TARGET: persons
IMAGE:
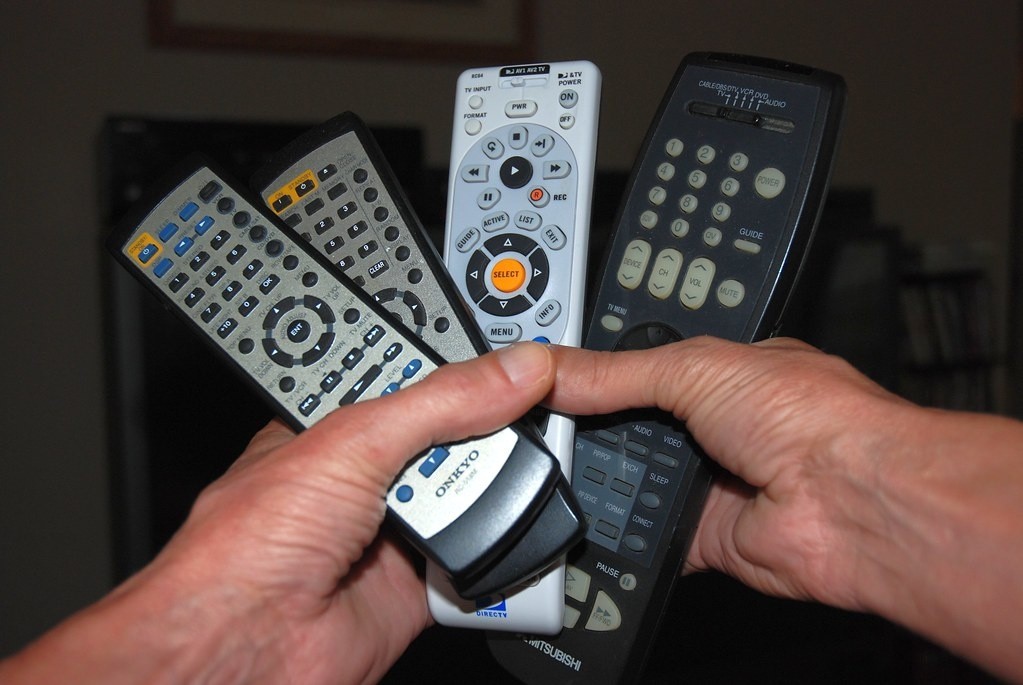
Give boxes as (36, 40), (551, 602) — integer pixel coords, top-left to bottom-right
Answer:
(0, 338), (1023, 685)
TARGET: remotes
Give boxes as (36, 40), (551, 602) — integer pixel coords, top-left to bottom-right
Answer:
(119, 50), (849, 685)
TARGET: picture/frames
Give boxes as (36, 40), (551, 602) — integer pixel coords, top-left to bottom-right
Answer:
(97, 116), (425, 584)
(146, 0), (541, 67)
